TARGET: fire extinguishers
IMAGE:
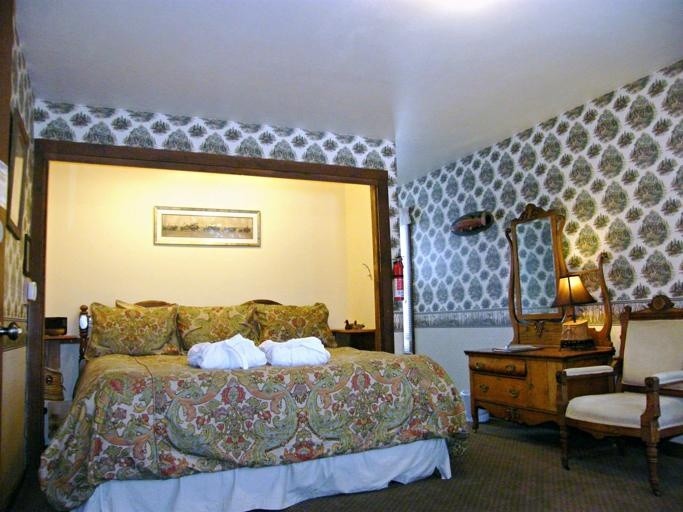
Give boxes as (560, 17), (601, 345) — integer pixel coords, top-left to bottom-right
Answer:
(392, 256), (404, 301)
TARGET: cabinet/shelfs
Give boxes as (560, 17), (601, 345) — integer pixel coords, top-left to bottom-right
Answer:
(463, 345), (615, 431)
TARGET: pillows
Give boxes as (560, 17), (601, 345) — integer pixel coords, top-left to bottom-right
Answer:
(113, 299), (177, 311)
(251, 300), (338, 347)
(82, 302), (181, 358)
(177, 299), (259, 351)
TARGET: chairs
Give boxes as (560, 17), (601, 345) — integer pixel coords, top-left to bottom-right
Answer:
(555, 293), (682, 496)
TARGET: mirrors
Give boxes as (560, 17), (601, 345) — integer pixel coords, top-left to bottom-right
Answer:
(510, 205), (563, 319)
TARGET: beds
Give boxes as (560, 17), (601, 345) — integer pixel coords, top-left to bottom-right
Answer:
(38, 299), (471, 510)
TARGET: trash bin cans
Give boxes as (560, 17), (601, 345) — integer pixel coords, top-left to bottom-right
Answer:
(461, 390), (490, 422)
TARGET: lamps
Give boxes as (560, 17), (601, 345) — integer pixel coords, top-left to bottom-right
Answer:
(550, 276), (597, 349)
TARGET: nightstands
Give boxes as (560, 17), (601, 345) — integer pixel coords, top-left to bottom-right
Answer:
(329, 327), (375, 350)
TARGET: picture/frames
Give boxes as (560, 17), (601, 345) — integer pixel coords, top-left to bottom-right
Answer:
(22, 234), (30, 276)
(5, 109), (30, 239)
(153, 205), (261, 247)
